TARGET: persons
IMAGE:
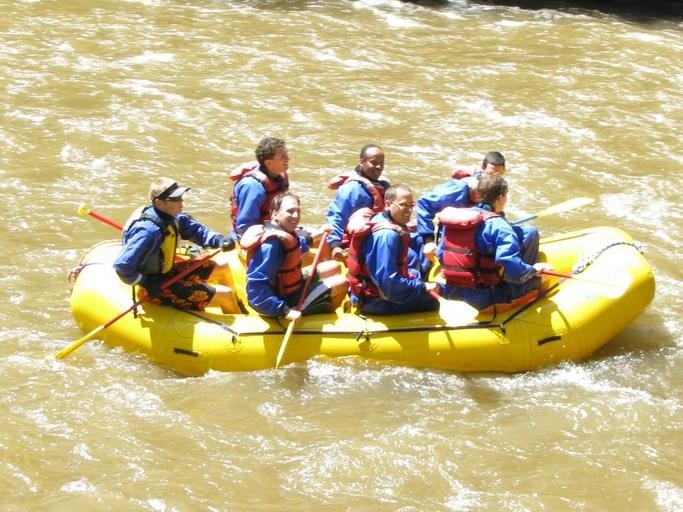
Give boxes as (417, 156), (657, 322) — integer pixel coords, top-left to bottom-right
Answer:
(346, 185), (439, 316)
(325, 145), (393, 261)
(247, 191), (336, 323)
(114, 177), (235, 310)
(230, 138), (290, 247)
(432, 175), (543, 310)
(417, 151), (540, 265)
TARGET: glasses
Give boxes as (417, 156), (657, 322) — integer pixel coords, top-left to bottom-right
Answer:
(392, 200), (416, 210)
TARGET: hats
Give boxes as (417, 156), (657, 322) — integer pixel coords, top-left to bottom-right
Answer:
(150, 177), (190, 201)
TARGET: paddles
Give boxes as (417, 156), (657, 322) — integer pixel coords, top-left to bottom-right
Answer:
(276, 225), (330, 370)
(427, 288), (479, 328)
(512, 197), (597, 225)
(539, 266), (635, 300)
(55, 234), (242, 360)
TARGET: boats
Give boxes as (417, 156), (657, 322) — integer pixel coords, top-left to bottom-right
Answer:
(66, 223), (657, 375)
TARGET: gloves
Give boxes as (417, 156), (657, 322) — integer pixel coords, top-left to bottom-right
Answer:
(140, 273), (165, 298)
(216, 237), (235, 252)
